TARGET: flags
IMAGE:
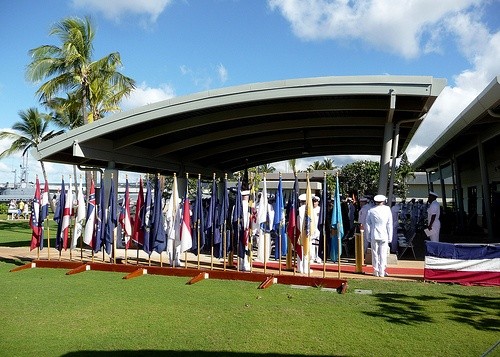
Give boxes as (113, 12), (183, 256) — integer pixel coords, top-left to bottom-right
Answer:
(53, 178), (74, 252)
(192, 178), (244, 259)
(248, 176), (344, 264)
(118, 177), (192, 266)
(29, 179), (49, 252)
(70, 178), (118, 254)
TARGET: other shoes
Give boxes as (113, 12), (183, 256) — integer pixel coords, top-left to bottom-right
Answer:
(240, 264), (250, 271)
(379, 273), (388, 277)
(375, 272), (379, 276)
(300, 269), (313, 273)
(171, 262), (183, 266)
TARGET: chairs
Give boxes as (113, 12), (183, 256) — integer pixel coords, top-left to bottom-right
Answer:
(396, 233), (417, 259)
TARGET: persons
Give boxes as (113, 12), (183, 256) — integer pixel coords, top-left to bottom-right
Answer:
(310, 196), (322, 264)
(252, 191), (275, 263)
(390, 199), (426, 253)
(51, 195), (57, 201)
(296, 193), (317, 273)
(7, 198), (28, 220)
(359, 194), (393, 277)
(425, 192), (440, 242)
(231, 190), (257, 272)
(341, 196), (355, 237)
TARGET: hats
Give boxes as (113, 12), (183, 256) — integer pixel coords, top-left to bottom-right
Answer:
(240, 190), (250, 196)
(298, 193), (307, 202)
(179, 198), (182, 204)
(314, 196), (320, 201)
(258, 192), (262, 196)
(374, 194), (386, 203)
(428, 191), (438, 197)
(360, 198), (367, 202)
